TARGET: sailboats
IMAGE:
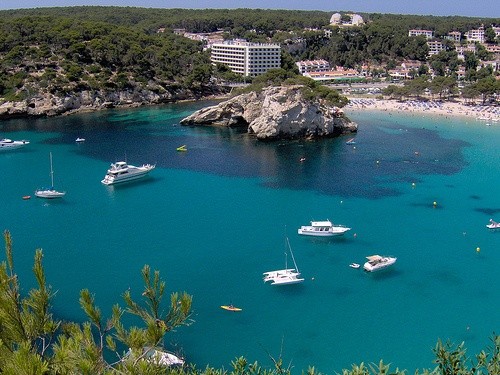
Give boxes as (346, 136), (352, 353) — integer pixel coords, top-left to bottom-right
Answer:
(260, 225), (305, 285)
(35, 152), (66, 198)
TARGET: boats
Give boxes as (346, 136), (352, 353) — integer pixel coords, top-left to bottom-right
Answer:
(299, 157), (306, 162)
(346, 137), (356, 145)
(101, 160), (156, 185)
(363, 254), (398, 272)
(22, 196), (31, 200)
(485, 224), (500, 229)
(120, 346), (188, 369)
(76, 137), (85, 141)
(221, 305), (243, 312)
(348, 262), (361, 269)
(296, 220), (351, 237)
(176, 145), (188, 151)
(0, 138), (30, 149)
